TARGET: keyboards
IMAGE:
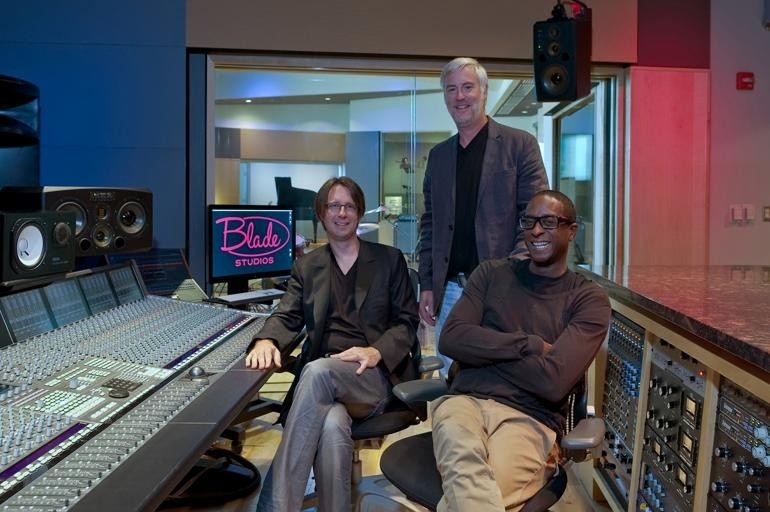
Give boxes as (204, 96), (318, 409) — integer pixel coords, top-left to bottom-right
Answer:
(216, 287), (286, 302)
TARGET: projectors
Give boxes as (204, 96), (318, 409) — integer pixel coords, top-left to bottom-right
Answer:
(532, 6), (592, 103)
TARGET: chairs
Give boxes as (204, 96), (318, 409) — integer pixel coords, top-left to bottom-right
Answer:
(274, 268), (443, 512)
(380, 374), (606, 512)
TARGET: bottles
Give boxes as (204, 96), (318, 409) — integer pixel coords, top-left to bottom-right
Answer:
(296, 233), (304, 261)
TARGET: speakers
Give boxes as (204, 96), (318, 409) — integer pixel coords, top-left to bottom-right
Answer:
(0, 211), (77, 282)
(41, 183), (155, 254)
(215, 126), (241, 158)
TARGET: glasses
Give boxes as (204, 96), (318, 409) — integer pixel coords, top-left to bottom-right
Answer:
(326, 203), (357, 212)
(518, 215), (572, 230)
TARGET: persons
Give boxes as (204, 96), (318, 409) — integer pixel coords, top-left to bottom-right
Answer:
(429, 190), (612, 512)
(418, 56), (551, 385)
(243, 175), (423, 511)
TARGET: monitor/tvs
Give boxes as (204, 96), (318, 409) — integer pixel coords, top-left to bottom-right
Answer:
(207, 204), (298, 295)
